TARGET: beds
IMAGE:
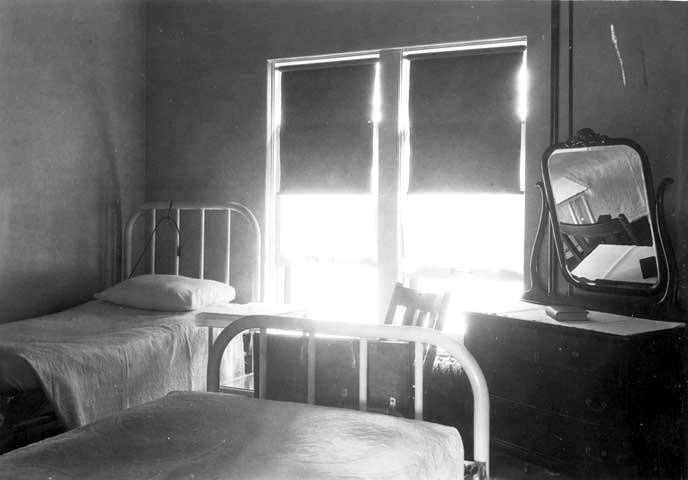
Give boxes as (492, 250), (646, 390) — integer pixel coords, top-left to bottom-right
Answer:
(0, 202), (260, 453)
(0, 314), (490, 480)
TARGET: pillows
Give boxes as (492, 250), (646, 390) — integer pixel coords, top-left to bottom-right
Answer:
(94, 273), (235, 312)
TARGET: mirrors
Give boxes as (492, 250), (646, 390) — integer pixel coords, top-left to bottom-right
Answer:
(519, 129), (674, 318)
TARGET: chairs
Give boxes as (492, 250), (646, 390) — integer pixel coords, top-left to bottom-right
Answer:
(378, 283), (445, 400)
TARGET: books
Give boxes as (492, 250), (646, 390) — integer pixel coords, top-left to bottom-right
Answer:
(640, 256), (658, 279)
(546, 305), (588, 321)
